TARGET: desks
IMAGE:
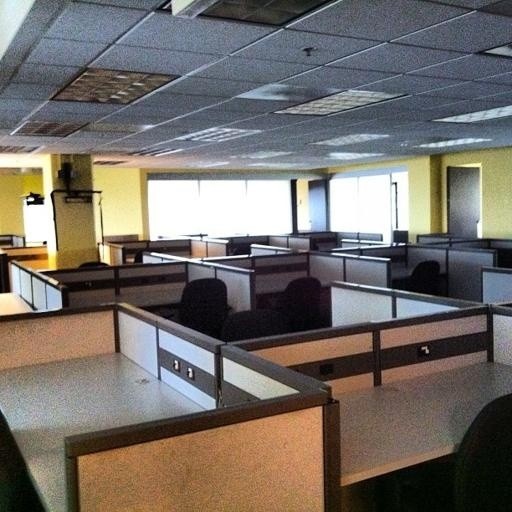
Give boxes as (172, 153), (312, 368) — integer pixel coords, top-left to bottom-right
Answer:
(0, 231), (512, 512)
